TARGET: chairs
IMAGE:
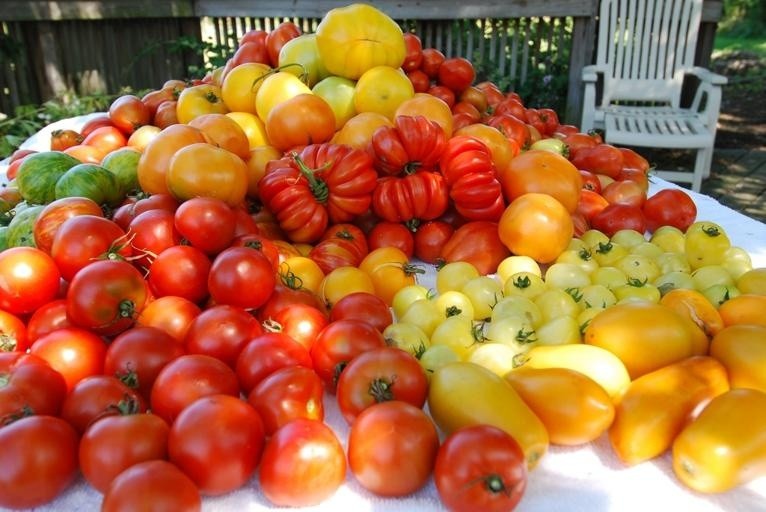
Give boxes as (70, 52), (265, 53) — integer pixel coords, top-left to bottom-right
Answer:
(581, 1), (729, 194)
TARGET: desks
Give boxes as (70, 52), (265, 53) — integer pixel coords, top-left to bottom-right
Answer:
(0, 110), (766, 511)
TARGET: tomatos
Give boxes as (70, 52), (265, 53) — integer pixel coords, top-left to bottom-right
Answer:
(0, 4), (766, 512)
